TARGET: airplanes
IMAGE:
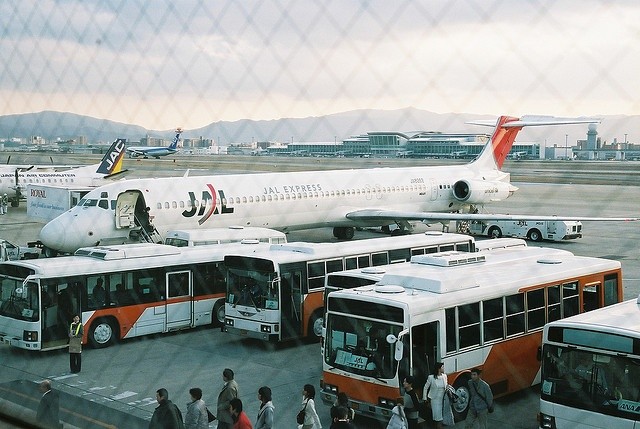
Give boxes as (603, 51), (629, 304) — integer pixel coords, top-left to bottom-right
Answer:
(40, 116), (640, 253)
(0, 139), (126, 207)
(125, 133), (180, 159)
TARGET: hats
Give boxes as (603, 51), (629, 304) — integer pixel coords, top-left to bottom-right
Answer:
(259, 385), (272, 400)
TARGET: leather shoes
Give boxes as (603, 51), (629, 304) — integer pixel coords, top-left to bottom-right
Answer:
(70, 369), (80, 373)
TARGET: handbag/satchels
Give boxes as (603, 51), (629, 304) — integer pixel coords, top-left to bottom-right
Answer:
(472, 382), (494, 413)
(205, 407), (217, 423)
(296, 396), (314, 424)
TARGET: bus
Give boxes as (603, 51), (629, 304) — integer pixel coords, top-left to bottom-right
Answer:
(539, 295), (640, 429)
(321, 246), (574, 357)
(0, 240), (278, 352)
(319, 255), (623, 427)
(163, 226), (287, 246)
(467, 215), (583, 243)
(224, 232), (476, 344)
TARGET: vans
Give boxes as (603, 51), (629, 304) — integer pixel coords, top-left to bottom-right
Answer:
(475, 238), (526, 252)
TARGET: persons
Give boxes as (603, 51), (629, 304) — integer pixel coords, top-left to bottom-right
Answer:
(216, 368), (238, 429)
(93, 277), (105, 302)
(295, 385), (323, 429)
(36, 382), (58, 427)
(115, 283), (123, 301)
(228, 398), (252, 428)
(3, 193), (8, 214)
(394, 375), (423, 429)
(330, 406), (354, 429)
(255, 387), (275, 429)
(148, 387), (183, 428)
(67, 316), (84, 373)
(183, 388), (209, 429)
(423, 362), (449, 429)
(0, 195), (3, 215)
(330, 392), (355, 429)
(139, 207), (151, 231)
(464, 369), (495, 428)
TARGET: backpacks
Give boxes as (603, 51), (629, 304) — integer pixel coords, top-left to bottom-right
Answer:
(348, 404), (355, 422)
